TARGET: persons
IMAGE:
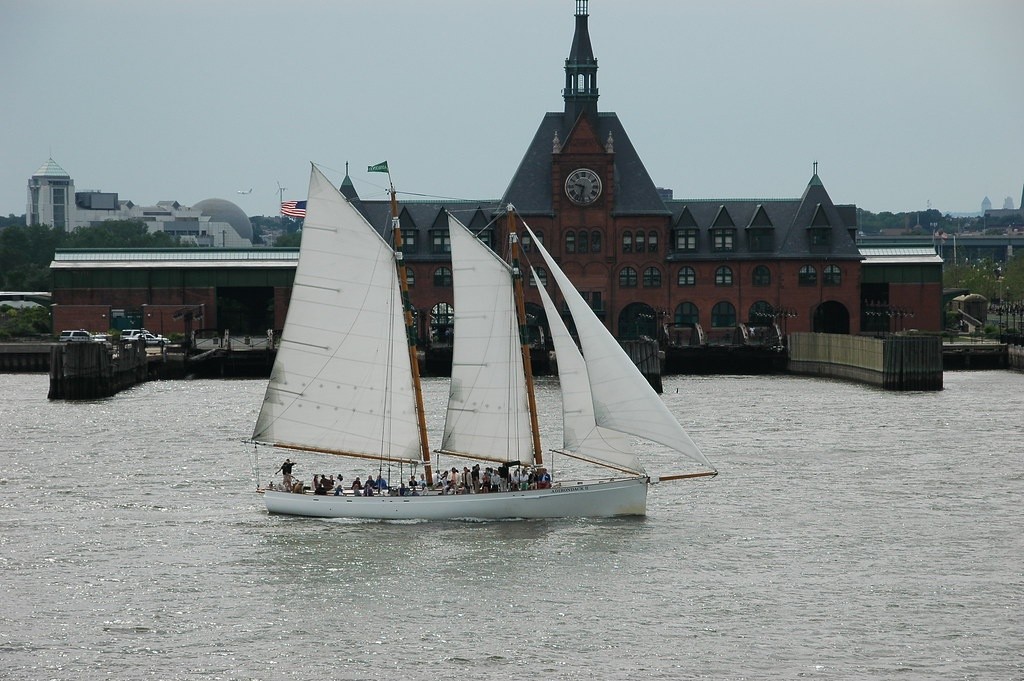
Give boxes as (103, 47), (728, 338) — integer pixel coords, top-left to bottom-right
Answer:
(429, 325), (454, 347)
(989, 302), (1024, 316)
(275, 459), (297, 493)
(311, 463), (552, 497)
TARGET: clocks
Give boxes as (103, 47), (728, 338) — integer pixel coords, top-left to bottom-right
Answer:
(565, 169), (602, 205)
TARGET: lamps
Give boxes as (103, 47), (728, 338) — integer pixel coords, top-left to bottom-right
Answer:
(147, 313), (152, 317)
(102, 314), (106, 317)
(49, 312), (54, 316)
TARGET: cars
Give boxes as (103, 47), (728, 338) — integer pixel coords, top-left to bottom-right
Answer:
(127, 333), (172, 347)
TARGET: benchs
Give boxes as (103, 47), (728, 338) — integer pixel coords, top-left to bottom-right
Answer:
(305, 487), (468, 496)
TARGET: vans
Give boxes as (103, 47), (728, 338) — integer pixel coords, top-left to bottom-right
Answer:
(119, 327), (151, 343)
(59, 329), (94, 344)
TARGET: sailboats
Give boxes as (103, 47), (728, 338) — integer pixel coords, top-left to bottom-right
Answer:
(250, 161), (719, 519)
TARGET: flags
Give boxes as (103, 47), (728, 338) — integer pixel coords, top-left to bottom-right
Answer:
(368, 162), (388, 174)
(280, 200), (308, 219)
